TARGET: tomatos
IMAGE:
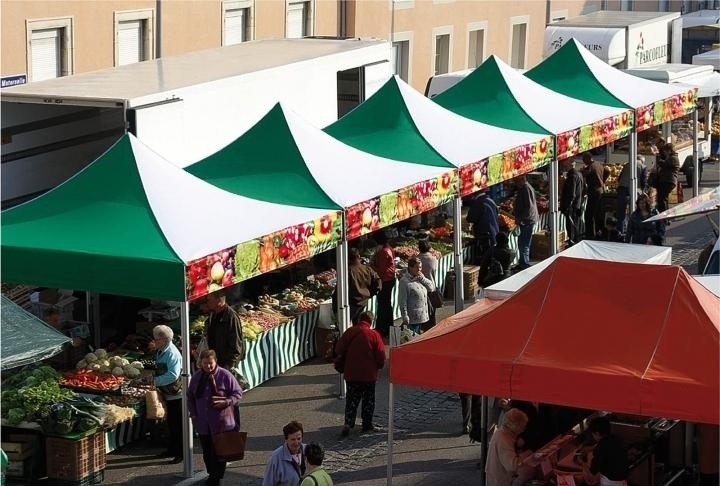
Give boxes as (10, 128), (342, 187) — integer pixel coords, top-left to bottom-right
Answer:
(279, 245), (288, 258)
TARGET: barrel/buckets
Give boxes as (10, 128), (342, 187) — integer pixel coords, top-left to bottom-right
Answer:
(313, 323), (339, 359)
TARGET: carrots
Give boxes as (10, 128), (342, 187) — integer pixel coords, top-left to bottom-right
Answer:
(62, 369), (127, 391)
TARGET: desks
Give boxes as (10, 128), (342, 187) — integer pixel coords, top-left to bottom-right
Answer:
(188, 286), (321, 390)
(285, 180), (587, 334)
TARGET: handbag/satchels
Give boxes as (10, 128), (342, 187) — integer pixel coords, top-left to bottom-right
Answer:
(211, 432), (248, 463)
(482, 247), (504, 284)
(427, 287), (444, 308)
(144, 376), (168, 420)
(334, 352), (346, 374)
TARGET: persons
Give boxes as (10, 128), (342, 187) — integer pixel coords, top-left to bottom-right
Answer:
(206, 291), (245, 432)
(399, 256), (435, 341)
(332, 248), (382, 325)
(698, 239), (715, 273)
(186, 349), (242, 485)
(418, 241), (437, 331)
(66, 335), (86, 369)
(334, 310), (386, 435)
(298, 442), (333, 486)
(697, 98), (707, 123)
(466, 138), (679, 287)
(458, 394), (627, 486)
(372, 229), (397, 343)
(714, 96), (720, 113)
(153, 325), (184, 464)
(263, 421), (307, 485)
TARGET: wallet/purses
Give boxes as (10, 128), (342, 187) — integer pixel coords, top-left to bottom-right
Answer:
(209, 395), (226, 407)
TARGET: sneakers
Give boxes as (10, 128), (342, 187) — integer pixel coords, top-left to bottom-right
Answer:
(160, 450), (183, 464)
(342, 425), (380, 438)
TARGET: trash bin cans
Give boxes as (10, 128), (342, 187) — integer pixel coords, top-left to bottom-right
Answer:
(683, 156), (704, 187)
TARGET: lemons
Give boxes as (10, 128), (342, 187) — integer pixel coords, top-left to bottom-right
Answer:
(644, 111), (650, 123)
(362, 208), (372, 228)
(567, 137), (574, 151)
(474, 170), (481, 186)
(211, 262), (224, 281)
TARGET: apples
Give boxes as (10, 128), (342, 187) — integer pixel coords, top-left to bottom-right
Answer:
(351, 222), (361, 231)
(193, 279), (207, 296)
(222, 269), (233, 287)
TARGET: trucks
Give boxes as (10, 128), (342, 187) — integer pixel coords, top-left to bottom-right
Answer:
(541, 7), (685, 67)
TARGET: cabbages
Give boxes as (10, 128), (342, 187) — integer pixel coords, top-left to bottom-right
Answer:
(76, 348), (144, 378)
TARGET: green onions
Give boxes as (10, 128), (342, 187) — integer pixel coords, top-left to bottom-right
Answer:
(63, 396), (136, 427)
